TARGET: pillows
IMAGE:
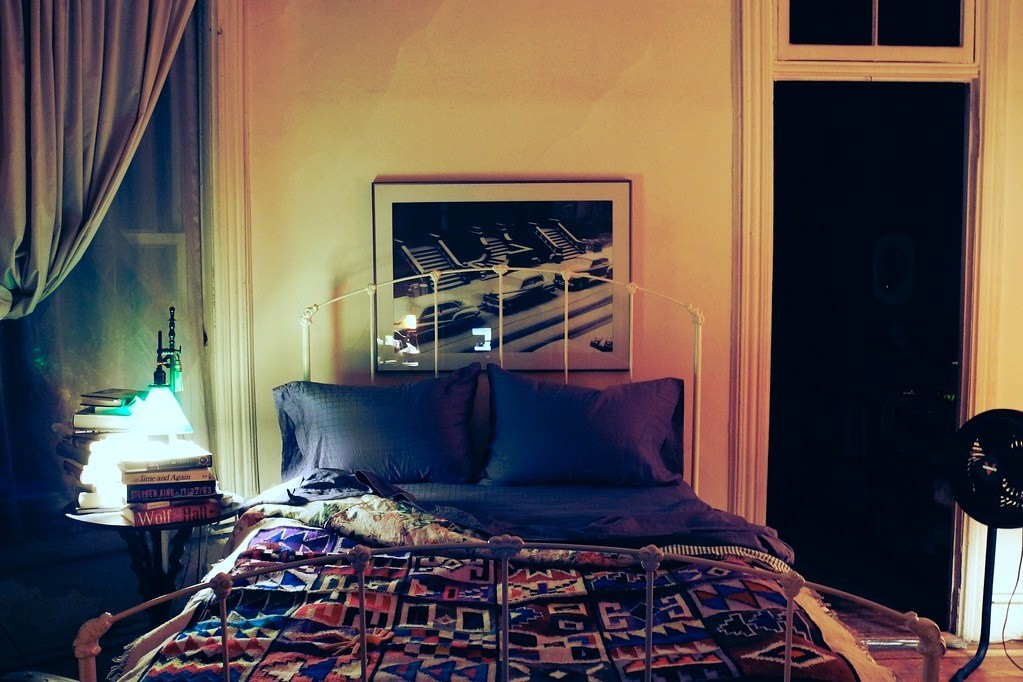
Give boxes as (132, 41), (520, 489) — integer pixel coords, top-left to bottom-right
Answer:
(480, 364), (684, 488)
(273, 362), (481, 484)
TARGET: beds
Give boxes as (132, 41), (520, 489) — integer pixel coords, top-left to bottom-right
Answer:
(72, 263), (947, 682)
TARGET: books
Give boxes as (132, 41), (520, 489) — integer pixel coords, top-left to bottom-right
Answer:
(56, 387), (240, 527)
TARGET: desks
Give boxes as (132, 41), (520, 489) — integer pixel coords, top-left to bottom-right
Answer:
(66, 491), (243, 631)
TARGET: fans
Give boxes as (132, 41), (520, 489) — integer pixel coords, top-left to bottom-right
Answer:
(948, 409), (1023, 682)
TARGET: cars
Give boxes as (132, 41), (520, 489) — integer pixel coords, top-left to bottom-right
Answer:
(395, 300), (481, 346)
(553, 256), (613, 292)
(482, 270), (554, 314)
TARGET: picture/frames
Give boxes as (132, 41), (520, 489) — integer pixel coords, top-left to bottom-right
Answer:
(372, 180), (633, 374)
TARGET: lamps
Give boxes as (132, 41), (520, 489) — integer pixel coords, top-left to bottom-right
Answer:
(130, 307), (194, 436)
